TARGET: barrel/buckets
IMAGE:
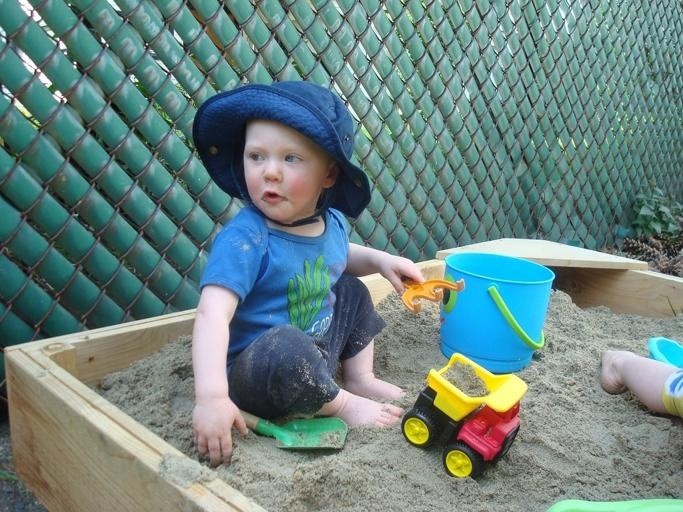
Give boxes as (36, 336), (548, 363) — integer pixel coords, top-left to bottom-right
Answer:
(439, 252), (558, 372)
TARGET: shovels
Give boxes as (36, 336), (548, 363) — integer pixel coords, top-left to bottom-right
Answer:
(230, 405), (348, 452)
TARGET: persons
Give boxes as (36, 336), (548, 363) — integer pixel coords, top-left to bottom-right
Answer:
(189, 83), (425, 466)
(597, 347), (682, 416)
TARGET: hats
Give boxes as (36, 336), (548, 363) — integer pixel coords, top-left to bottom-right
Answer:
(192, 80), (371, 218)
